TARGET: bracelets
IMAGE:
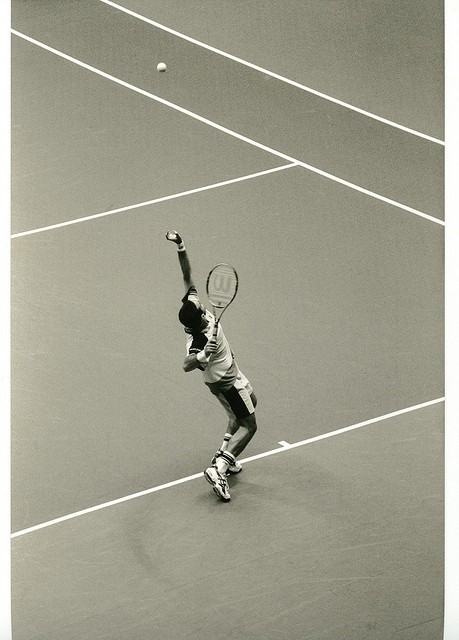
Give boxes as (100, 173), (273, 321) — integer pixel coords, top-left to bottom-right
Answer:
(177, 241), (184, 248)
(197, 350), (207, 363)
(176, 247), (185, 252)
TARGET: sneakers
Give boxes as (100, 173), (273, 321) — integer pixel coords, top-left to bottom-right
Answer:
(205, 466), (231, 502)
(211, 449), (242, 472)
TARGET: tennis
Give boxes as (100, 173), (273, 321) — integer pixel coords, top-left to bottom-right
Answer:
(157, 63), (166, 72)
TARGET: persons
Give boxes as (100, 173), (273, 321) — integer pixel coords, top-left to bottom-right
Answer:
(164, 230), (257, 501)
